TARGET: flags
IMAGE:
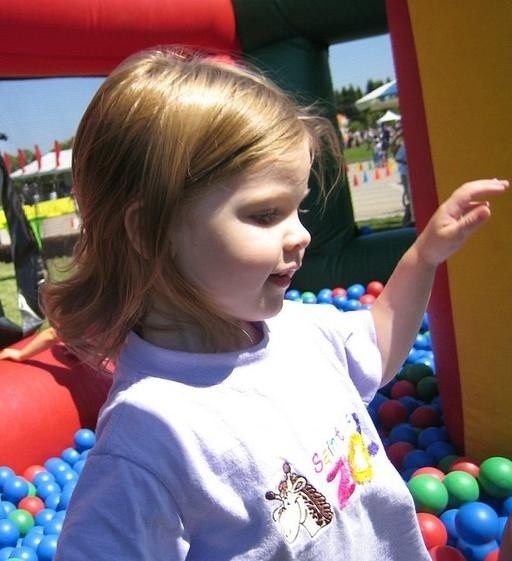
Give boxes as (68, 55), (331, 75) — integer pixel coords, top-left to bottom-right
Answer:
(18, 147), (26, 173)
(55, 139), (61, 167)
(34, 144), (42, 170)
(4, 151), (12, 176)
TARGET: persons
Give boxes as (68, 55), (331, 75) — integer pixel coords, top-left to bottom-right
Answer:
(343, 125), (411, 227)
(36, 42), (512, 561)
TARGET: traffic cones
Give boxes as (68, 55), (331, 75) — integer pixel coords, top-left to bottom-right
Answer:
(345, 159), (397, 187)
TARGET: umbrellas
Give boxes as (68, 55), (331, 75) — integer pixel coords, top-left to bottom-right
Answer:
(355, 79), (400, 112)
(375, 109), (401, 123)
